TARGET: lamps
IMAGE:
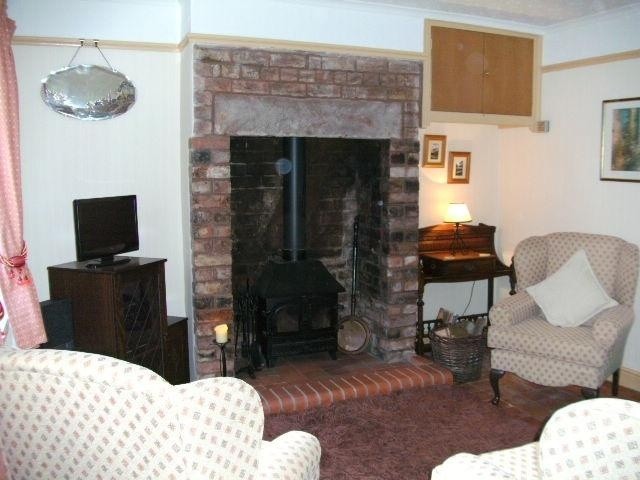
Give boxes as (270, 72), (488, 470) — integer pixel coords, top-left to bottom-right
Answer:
(443, 202), (473, 256)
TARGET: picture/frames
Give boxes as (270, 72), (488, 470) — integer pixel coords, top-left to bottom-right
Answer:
(448, 151), (471, 184)
(600, 97), (640, 183)
(422, 134), (447, 168)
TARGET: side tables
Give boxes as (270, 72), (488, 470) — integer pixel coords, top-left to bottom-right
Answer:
(416, 223), (517, 357)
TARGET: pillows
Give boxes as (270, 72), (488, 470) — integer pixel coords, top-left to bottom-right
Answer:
(525, 248), (620, 329)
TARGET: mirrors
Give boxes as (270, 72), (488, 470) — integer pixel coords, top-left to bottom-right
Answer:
(41, 63), (136, 120)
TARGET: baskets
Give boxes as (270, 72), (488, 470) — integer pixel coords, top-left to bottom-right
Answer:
(428, 324), (487, 384)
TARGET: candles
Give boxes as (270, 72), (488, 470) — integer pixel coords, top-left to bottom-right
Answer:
(213, 324), (229, 344)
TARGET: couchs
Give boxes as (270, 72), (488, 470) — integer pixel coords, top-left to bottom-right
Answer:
(487, 232), (639, 405)
(431, 398), (640, 480)
(0, 349), (323, 480)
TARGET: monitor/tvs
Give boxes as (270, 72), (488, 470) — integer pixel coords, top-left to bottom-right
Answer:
(73, 195), (140, 269)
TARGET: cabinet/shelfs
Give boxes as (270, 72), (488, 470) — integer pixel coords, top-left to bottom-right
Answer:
(47, 257), (190, 385)
(422, 18), (543, 128)
